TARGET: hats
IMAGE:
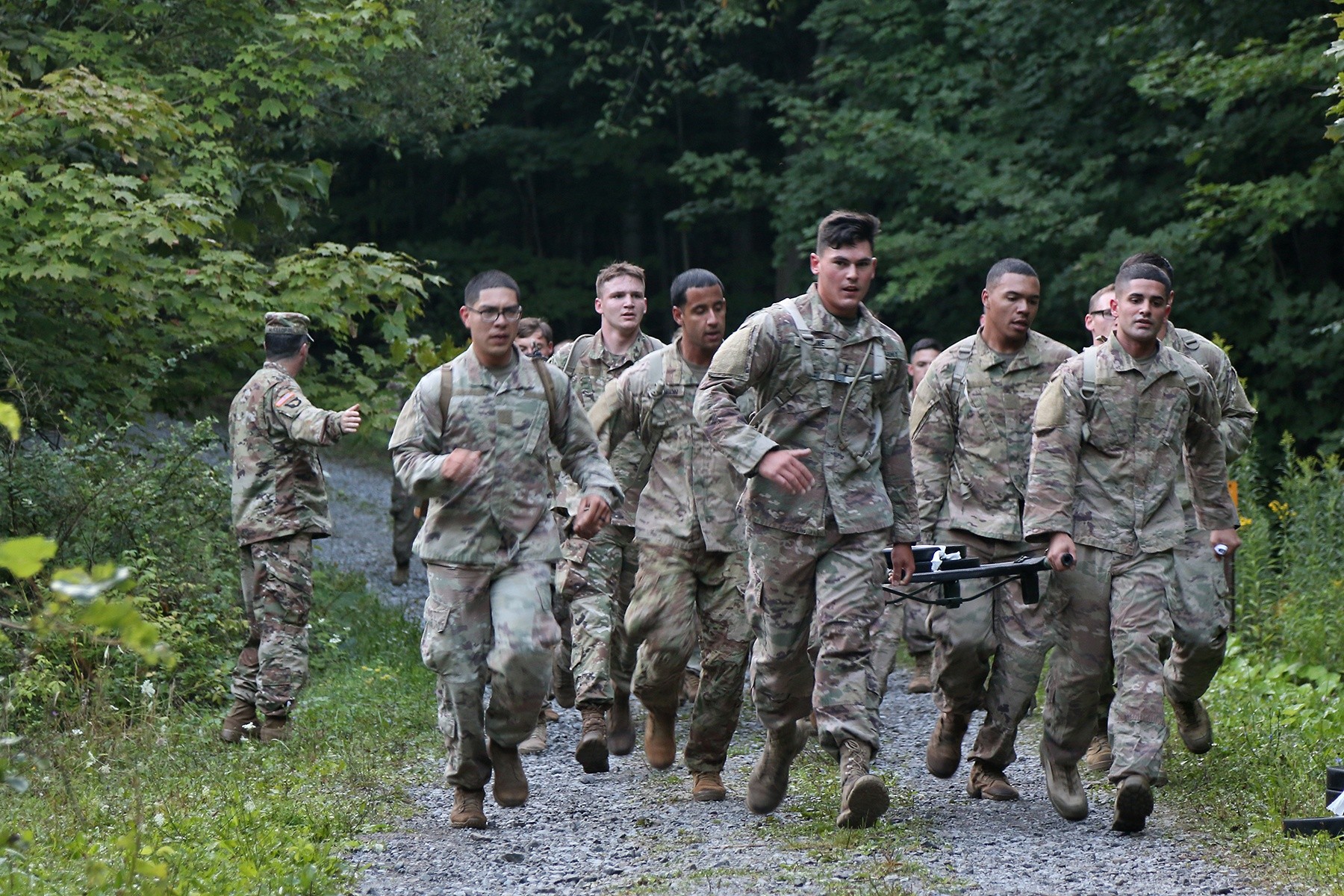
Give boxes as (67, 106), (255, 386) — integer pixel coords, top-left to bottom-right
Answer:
(265, 311), (314, 342)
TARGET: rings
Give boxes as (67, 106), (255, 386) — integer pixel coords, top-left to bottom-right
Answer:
(600, 517), (605, 522)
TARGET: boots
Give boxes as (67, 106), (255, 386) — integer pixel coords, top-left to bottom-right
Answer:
(607, 701), (636, 756)
(966, 762), (1020, 800)
(488, 738), (528, 807)
(261, 716), (322, 743)
(1164, 681), (1213, 754)
(927, 712), (971, 778)
(1040, 750), (1088, 823)
(836, 739), (889, 829)
(222, 700), (264, 743)
(1087, 722), (1114, 772)
(518, 711), (547, 753)
(574, 706), (610, 773)
(907, 650), (934, 693)
(451, 786), (487, 829)
(1111, 773), (1154, 833)
(551, 645), (576, 708)
(747, 720), (808, 814)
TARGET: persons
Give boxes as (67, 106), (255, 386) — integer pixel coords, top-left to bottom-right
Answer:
(386, 210), (1259, 833)
(220, 311), (362, 744)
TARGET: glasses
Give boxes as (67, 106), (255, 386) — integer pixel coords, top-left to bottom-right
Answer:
(466, 306), (522, 322)
(1090, 309), (1115, 320)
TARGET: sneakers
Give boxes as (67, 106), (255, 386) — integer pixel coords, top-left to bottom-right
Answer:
(685, 672), (699, 703)
(692, 772), (726, 801)
(645, 710), (676, 769)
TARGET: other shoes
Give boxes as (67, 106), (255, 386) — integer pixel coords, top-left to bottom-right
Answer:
(392, 565), (410, 584)
(804, 714), (817, 736)
